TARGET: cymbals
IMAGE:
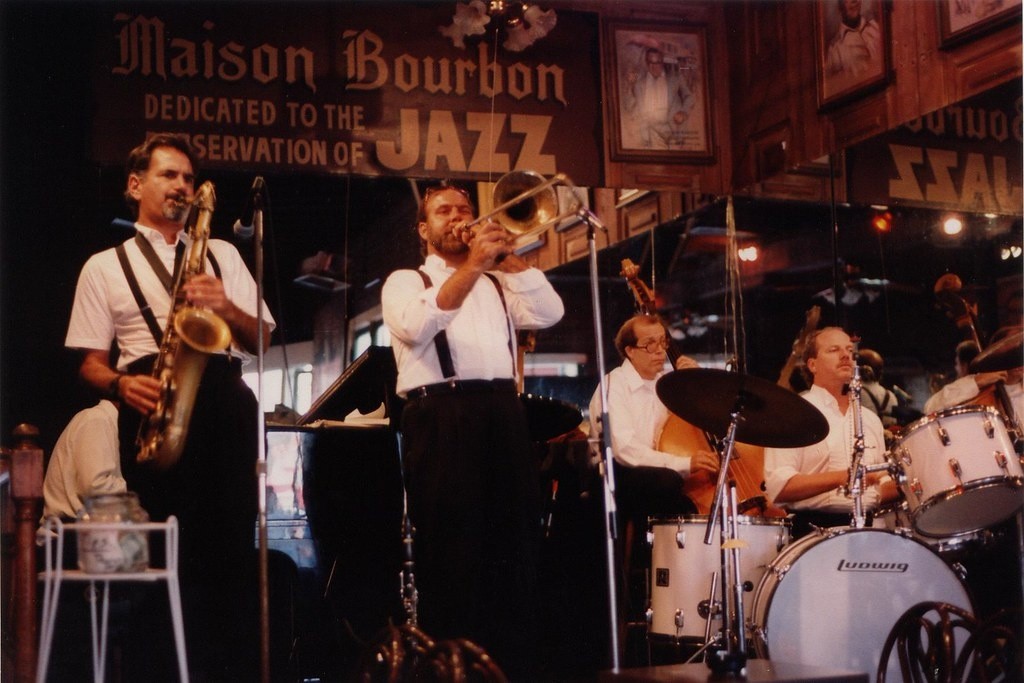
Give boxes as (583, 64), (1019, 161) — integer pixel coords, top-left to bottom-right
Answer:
(655, 367), (830, 449)
(971, 330), (1024, 364)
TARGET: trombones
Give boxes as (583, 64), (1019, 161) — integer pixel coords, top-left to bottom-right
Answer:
(459, 169), (583, 253)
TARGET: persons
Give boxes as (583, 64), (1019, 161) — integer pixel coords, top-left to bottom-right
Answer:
(382, 180), (566, 683)
(763, 326), (900, 541)
(63, 131), (276, 683)
(588, 316), (720, 611)
(855, 348), (899, 417)
(922, 325), (1024, 451)
(954, 340), (979, 377)
(36, 390), (154, 683)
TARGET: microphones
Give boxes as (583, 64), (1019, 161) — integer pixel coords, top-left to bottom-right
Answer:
(233, 176), (263, 239)
(569, 200), (607, 234)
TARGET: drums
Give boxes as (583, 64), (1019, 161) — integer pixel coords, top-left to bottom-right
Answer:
(750, 524), (981, 683)
(885, 400), (1024, 543)
(646, 514), (791, 642)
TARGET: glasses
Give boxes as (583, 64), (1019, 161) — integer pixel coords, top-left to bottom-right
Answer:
(424, 186), (471, 207)
(633, 338), (670, 354)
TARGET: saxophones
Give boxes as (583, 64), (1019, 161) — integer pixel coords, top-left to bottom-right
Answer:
(133, 181), (231, 464)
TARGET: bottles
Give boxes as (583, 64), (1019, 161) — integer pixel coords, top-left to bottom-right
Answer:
(75, 493), (150, 575)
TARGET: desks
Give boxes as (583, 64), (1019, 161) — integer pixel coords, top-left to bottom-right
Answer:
(34, 516), (192, 683)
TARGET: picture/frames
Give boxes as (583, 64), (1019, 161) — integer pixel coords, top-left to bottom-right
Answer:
(810, 0), (893, 114)
(613, 188), (651, 209)
(934, 0), (1023, 50)
(512, 232), (546, 255)
(601, 15), (718, 168)
(551, 185), (593, 232)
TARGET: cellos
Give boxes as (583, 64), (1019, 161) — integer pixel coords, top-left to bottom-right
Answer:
(621, 257), (787, 518)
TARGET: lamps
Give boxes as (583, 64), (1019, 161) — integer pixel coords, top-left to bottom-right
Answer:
(453, 0), (556, 52)
